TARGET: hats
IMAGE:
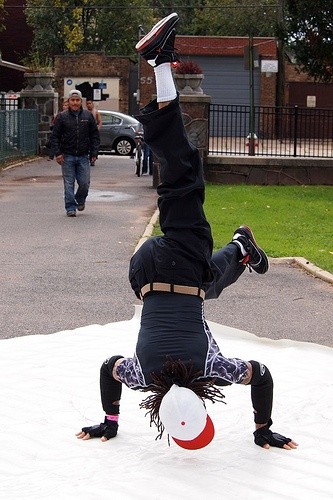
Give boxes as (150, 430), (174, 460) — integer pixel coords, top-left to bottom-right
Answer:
(158, 384), (215, 451)
(67, 89), (83, 101)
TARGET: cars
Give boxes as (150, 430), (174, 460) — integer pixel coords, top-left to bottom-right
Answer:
(96, 110), (144, 156)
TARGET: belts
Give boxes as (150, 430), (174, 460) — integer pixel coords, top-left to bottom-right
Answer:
(138, 283), (206, 301)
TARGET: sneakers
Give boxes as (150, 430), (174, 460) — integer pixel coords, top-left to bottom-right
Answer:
(135, 12), (182, 68)
(230, 225), (270, 275)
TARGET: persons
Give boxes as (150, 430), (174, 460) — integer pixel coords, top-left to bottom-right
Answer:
(75, 12), (297, 450)
(85, 98), (101, 167)
(137, 136), (154, 177)
(50, 100), (68, 128)
(52, 90), (100, 217)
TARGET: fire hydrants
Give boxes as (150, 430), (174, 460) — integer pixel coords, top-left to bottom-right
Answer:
(246, 133), (258, 156)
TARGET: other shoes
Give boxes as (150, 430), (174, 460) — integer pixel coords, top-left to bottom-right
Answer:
(48, 158), (52, 161)
(77, 202), (85, 211)
(90, 161), (96, 166)
(66, 210), (77, 217)
